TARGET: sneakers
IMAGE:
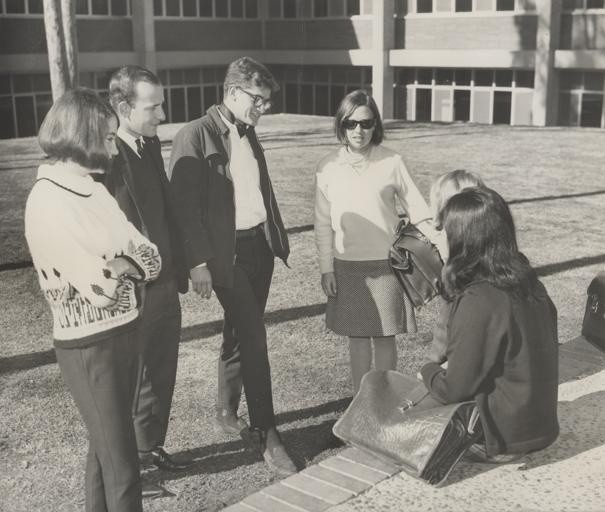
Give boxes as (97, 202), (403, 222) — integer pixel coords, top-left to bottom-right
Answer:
(249, 426), (297, 476)
(213, 415), (251, 437)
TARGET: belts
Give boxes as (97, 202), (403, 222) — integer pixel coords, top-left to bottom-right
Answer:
(235, 225), (265, 240)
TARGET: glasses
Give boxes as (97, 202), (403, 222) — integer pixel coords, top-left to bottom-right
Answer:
(344, 118), (377, 130)
(236, 86), (273, 109)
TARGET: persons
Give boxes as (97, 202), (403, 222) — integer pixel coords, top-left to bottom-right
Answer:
(414, 185), (562, 464)
(414, 168), (482, 382)
(24, 86), (164, 512)
(166, 55), (299, 477)
(89, 64), (191, 483)
(312, 89), (434, 399)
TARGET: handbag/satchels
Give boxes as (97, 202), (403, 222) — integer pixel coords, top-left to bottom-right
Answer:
(389, 220), (445, 311)
(333, 366), (485, 489)
(583, 270), (604, 352)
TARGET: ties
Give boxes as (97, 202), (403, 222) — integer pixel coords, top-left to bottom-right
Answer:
(135, 139), (144, 157)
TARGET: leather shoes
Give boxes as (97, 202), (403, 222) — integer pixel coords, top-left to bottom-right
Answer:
(140, 481), (165, 500)
(137, 448), (195, 471)
